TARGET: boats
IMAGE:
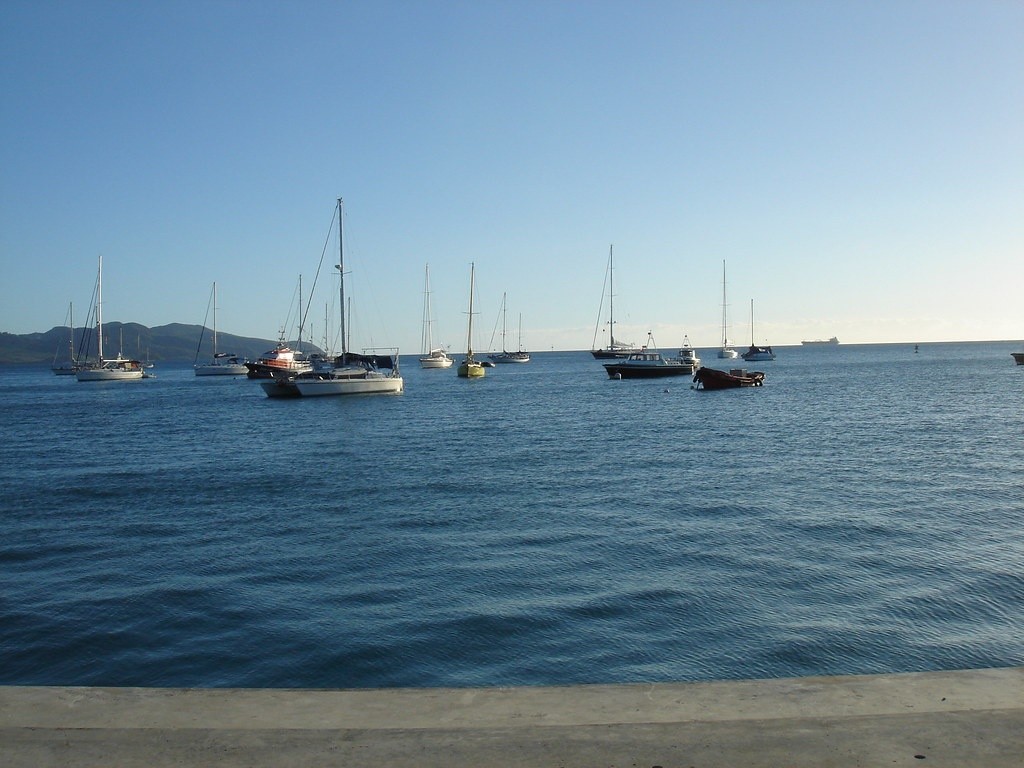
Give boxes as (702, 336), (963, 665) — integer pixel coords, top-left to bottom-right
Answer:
(693, 366), (765, 390)
(481, 361), (496, 367)
(674, 336), (701, 369)
(602, 329), (694, 380)
(1010, 352), (1024, 365)
(801, 337), (839, 345)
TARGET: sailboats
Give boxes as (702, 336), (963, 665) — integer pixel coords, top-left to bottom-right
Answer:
(51, 301), (80, 376)
(191, 281), (248, 376)
(418, 262), (453, 369)
(244, 274), (334, 379)
(259, 198), (404, 396)
(588, 243), (646, 359)
(457, 261), (485, 379)
(740, 299), (778, 361)
(75, 254), (146, 381)
(718, 259), (738, 359)
(486, 292), (530, 364)
(115, 327), (155, 369)
